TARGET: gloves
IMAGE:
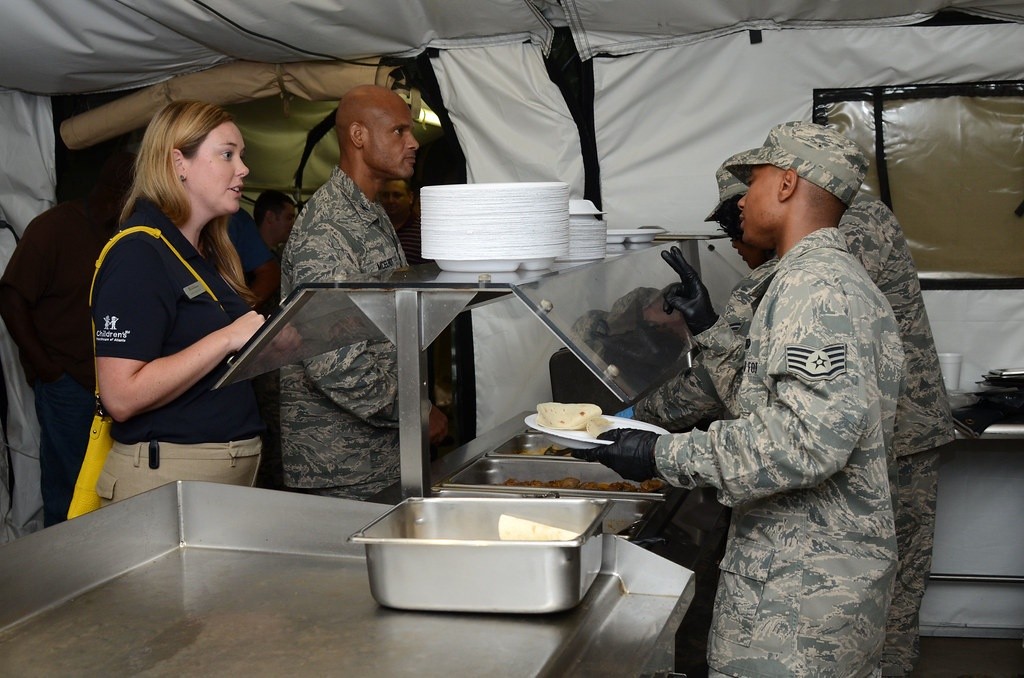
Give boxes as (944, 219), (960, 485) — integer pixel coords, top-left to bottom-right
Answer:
(660, 245), (720, 337)
(571, 428), (664, 482)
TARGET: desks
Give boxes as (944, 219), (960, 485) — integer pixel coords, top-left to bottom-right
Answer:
(927, 425), (1024, 584)
(0, 479), (695, 678)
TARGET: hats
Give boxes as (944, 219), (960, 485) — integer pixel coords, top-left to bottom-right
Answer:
(703, 145), (765, 223)
(722, 120), (872, 211)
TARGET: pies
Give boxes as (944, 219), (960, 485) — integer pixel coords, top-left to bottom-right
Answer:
(499, 514), (580, 542)
(536, 403), (602, 430)
(587, 417), (639, 438)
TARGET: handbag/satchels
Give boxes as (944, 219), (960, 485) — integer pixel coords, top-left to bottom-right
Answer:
(66, 413), (115, 521)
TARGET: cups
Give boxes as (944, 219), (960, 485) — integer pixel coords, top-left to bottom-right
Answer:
(934, 352), (962, 390)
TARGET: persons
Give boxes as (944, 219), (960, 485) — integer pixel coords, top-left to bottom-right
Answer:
(91, 99), (302, 508)
(570, 121), (907, 678)
(612, 146), (781, 431)
(574, 284), (691, 393)
(277, 84), (447, 502)
(840, 188), (955, 677)
(0, 152), (422, 530)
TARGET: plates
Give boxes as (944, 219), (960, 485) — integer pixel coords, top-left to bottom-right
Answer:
(525, 413), (669, 450)
(418, 182), (667, 274)
